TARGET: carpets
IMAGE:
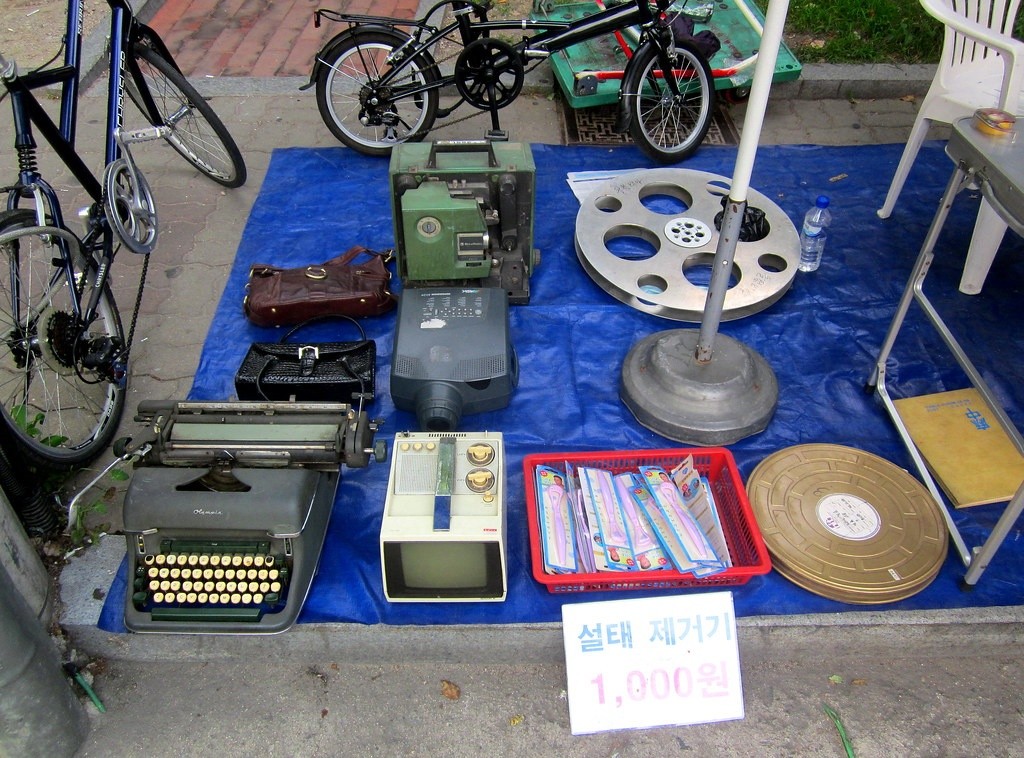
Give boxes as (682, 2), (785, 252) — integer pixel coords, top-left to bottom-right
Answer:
(94, 137), (1023, 634)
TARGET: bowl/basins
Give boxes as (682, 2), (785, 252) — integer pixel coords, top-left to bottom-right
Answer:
(975, 108), (1016, 135)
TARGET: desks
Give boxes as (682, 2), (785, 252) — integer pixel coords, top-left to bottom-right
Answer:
(862, 114), (1022, 594)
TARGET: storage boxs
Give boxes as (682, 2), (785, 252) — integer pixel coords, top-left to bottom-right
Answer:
(522, 446), (773, 595)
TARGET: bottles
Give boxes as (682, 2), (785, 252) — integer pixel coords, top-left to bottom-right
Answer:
(798, 197), (832, 272)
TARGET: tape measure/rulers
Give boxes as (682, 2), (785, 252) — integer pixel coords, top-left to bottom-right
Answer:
(972, 107), (1018, 143)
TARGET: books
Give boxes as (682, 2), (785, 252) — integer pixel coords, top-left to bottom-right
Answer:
(891, 388), (1024, 508)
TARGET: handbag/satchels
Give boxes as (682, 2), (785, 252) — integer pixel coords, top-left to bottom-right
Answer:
(244, 245), (399, 328)
(234, 312), (376, 406)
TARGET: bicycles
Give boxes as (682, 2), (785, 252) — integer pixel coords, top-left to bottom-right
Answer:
(304, 1), (724, 164)
(0, 0), (249, 474)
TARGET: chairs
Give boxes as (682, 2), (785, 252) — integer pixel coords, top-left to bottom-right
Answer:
(877, 0), (1024, 297)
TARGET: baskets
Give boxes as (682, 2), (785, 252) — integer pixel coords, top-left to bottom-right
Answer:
(522, 446), (770, 594)
